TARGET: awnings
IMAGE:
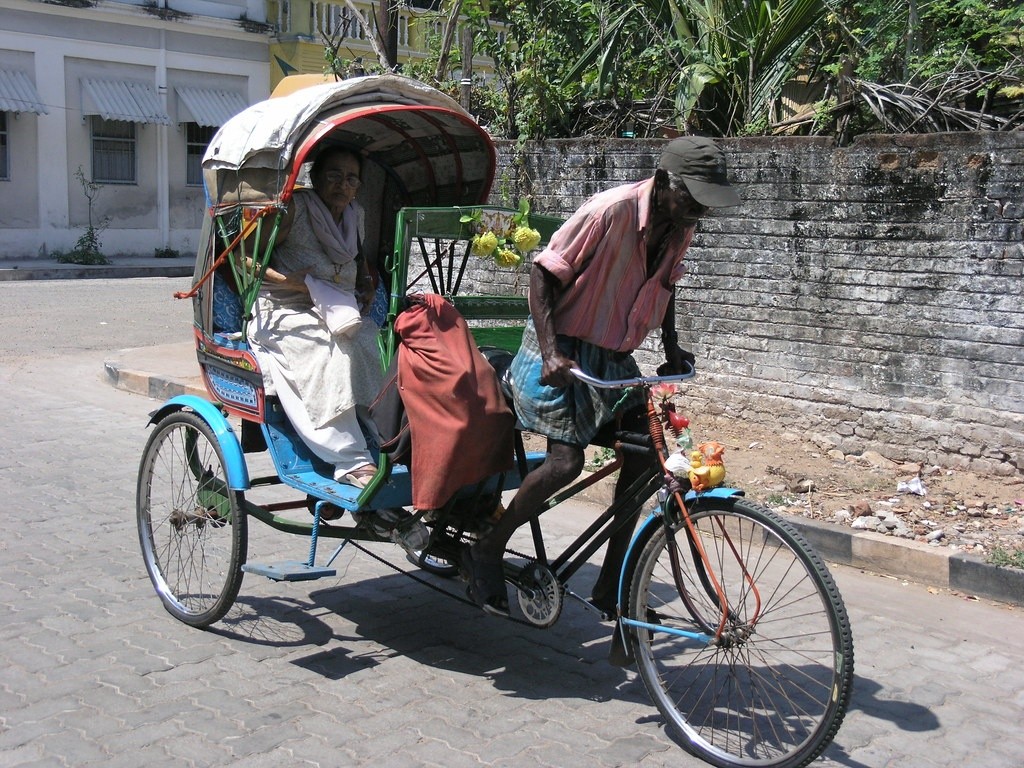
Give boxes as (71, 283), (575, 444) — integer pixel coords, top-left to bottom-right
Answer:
(79, 74), (175, 129)
(174, 85), (251, 130)
(1, 69), (47, 116)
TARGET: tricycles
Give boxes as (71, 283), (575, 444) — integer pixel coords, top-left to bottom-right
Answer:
(135, 71), (857, 768)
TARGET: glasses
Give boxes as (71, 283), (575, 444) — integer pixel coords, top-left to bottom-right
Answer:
(324, 171), (362, 190)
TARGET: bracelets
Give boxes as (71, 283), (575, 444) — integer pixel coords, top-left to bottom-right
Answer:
(661, 331), (678, 344)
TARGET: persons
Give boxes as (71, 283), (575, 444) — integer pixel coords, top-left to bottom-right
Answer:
(456, 137), (745, 634)
(228, 146), (401, 490)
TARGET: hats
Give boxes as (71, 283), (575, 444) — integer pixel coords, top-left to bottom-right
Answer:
(659, 136), (743, 208)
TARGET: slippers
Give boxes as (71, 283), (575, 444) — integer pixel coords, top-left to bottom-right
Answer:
(592, 586), (661, 630)
(336, 469), (377, 489)
(447, 542), (510, 618)
